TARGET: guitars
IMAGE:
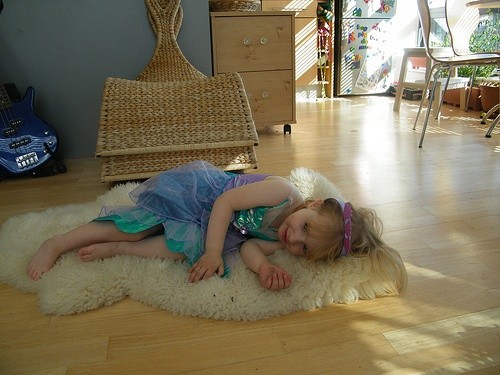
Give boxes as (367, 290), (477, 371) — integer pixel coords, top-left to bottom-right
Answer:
(0, 79), (60, 177)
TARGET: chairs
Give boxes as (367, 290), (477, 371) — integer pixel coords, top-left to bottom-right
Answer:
(413, 0), (500, 148)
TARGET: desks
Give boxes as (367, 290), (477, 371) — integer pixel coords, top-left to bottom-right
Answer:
(465, 0), (500, 138)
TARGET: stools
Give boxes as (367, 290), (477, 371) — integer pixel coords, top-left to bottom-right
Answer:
(394, 0), (470, 116)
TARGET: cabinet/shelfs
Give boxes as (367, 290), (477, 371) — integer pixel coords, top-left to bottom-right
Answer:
(210, 10), (297, 135)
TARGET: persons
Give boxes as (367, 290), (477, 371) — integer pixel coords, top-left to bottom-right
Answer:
(26, 159), (384, 291)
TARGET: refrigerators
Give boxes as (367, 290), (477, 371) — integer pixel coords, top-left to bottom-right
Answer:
(335, 0), (397, 96)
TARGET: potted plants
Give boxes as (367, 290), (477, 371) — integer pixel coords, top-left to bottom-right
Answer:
(439, 57), (485, 112)
(468, 6), (500, 113)
